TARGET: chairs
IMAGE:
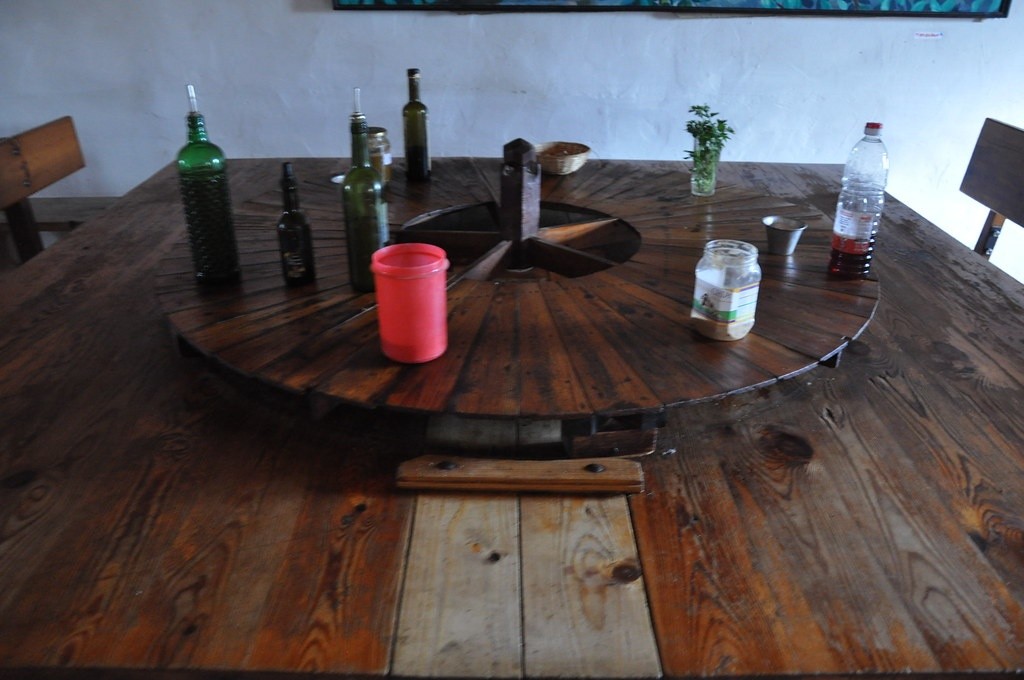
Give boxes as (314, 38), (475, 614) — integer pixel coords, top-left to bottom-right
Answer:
(961, 119), (1024, 259)
(0, 115), (87, 257)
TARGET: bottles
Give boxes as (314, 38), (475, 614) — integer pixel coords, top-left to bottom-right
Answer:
(368, 127), (392, 195)
(178, 84), (238, 282)
(276, 162), (316, 286)
(690, 238), (763, 342)
(402, 69), (432, 182)
(829, 122), (889, 276)
(342, 88), (389, 292)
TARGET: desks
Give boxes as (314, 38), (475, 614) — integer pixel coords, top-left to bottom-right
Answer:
(0, 155), (1023, 679)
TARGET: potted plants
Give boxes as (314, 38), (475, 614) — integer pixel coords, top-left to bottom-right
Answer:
(684, 106), (736, 195)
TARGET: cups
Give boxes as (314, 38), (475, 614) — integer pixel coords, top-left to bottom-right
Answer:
(761, 216), (808, 256)
(369, 243), (450, 363)
